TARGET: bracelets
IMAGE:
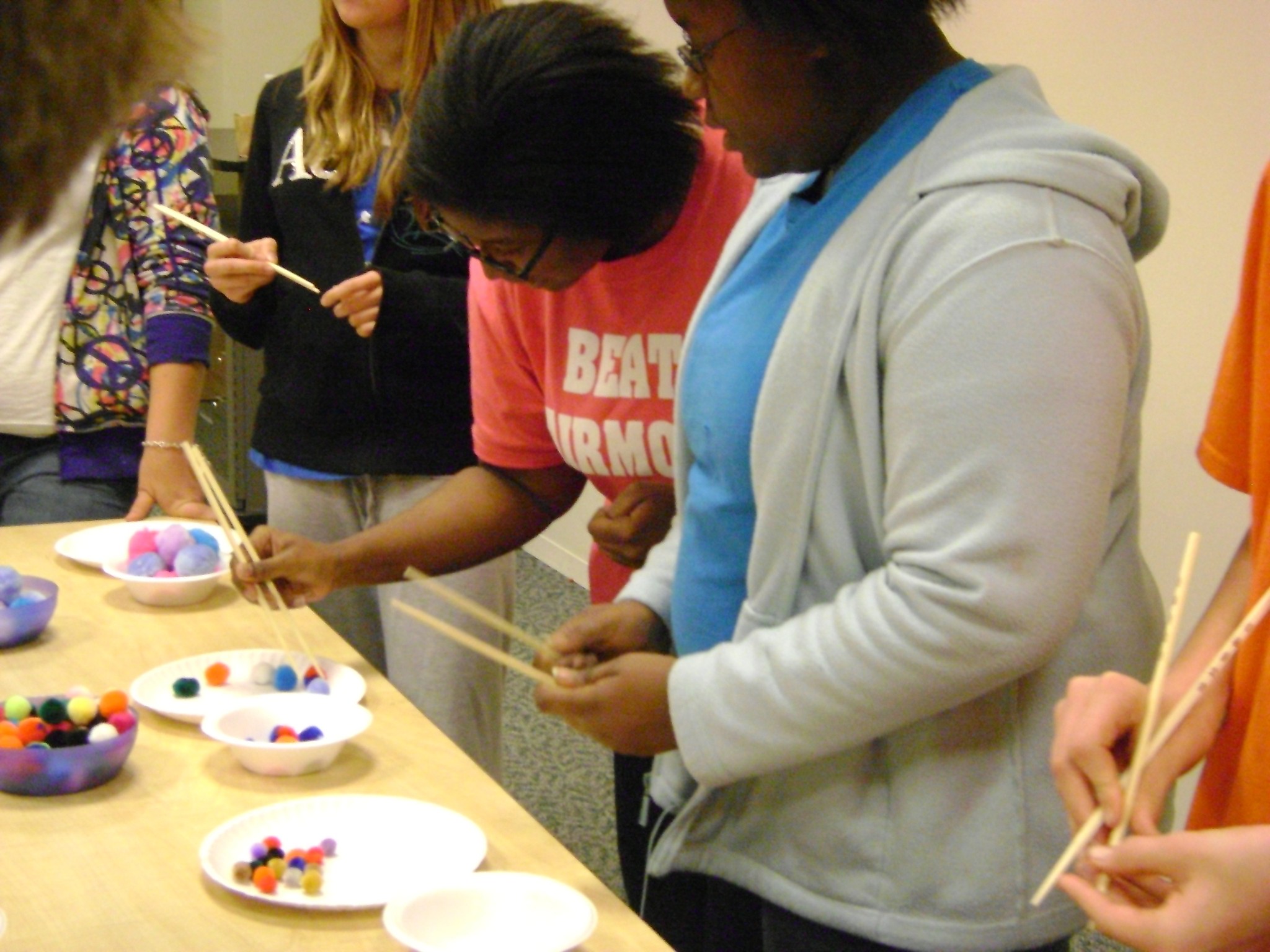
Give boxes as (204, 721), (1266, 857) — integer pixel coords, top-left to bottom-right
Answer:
(143, 441), (184, 451)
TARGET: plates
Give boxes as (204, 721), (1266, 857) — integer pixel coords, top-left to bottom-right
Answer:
(128, 649), (366, 724)
(54, 519), (243, 570)
(199, 794), (486, 907)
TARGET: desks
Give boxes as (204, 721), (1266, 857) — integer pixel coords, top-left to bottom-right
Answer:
(0, 514), (678, 951)
(204, 127), (246, 510)
(521, 480), (604, 590)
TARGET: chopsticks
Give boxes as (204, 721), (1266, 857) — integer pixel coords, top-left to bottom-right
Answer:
(152, 201), (317, 295)
(182, 437), (329, 684)
(391, 562), (563, 693)
(1031, 531), (1270, 931)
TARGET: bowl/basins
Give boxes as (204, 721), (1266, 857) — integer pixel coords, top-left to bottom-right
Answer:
(101, 548), (234, 605)
(0, 695), (139, 796)
(0, 576), (57, 647)
(382, 869), (597, 952)
(200, 693), (374, 776)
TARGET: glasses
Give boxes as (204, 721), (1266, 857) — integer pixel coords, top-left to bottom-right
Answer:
(677, 23), (750, 75)
(424, 210), (561, 281)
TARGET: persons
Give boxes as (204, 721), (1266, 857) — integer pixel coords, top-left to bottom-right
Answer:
(0, 0), (218, 526)
(530, 0), (1172, 952)
(227, 0), (756, 917)
(1051, 162), (1270, 952)
(202, 0), (514, 782)
